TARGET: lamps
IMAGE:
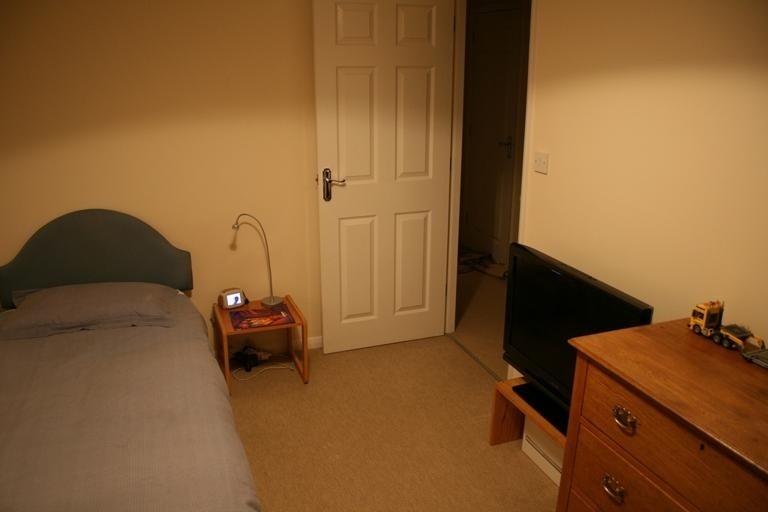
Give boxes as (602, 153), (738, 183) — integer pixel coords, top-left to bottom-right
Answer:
(232, 212), (284, 307)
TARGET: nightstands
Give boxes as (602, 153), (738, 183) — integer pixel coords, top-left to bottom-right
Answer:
(211, 293), (310, 396)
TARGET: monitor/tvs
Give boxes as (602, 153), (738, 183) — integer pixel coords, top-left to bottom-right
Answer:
(502, 243), (654, 440)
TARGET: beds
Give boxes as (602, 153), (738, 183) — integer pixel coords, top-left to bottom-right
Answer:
(0, 207), (259, 510)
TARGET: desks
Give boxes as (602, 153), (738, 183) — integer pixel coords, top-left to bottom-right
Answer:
(486, 376), (570, 446)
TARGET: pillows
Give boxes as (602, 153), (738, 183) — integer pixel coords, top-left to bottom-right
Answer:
(3, 280), (182, 339)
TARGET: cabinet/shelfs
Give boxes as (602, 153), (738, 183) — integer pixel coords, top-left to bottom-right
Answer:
(551, 313), (768, 512)
